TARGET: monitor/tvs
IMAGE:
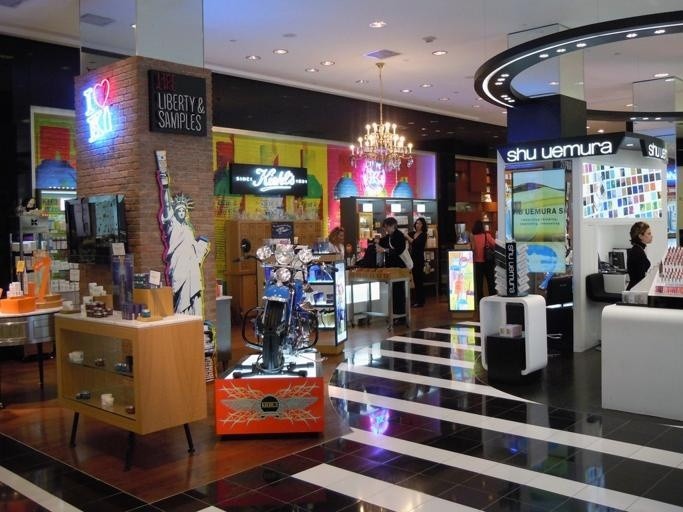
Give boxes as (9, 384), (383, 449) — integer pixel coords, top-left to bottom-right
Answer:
(65, 193), (129, 265)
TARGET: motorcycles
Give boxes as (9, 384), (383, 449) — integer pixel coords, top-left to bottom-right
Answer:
(236, 243), (339, 370)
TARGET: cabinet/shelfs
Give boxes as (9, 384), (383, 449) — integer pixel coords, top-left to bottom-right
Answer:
(54, 312), (209, 471)
(339, 196), (442, 297)
(256, 260), (345, 356)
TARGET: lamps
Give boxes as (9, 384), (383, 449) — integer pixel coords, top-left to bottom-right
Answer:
(348, 62), (415, 171)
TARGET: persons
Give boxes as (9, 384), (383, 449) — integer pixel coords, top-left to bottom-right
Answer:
(402, 218), (429, 307)
(327, 227), (345, 255)
(472, 220), (496, 304)
(345, 240), (357, 267)
(625, 221), (654, 291)
(158, 171), (209, 320)
(375, 217), (407, 325)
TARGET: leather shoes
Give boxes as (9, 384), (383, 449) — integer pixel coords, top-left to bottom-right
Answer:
(412, 302), (422, 307)
(385, 319), (406, 325)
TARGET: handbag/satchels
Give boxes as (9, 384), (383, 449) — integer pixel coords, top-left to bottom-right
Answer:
(399, 249), (414, 270)
(484, 246), (496, 268)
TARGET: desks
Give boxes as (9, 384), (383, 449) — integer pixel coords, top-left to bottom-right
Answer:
(350, 276), (412, 333)
